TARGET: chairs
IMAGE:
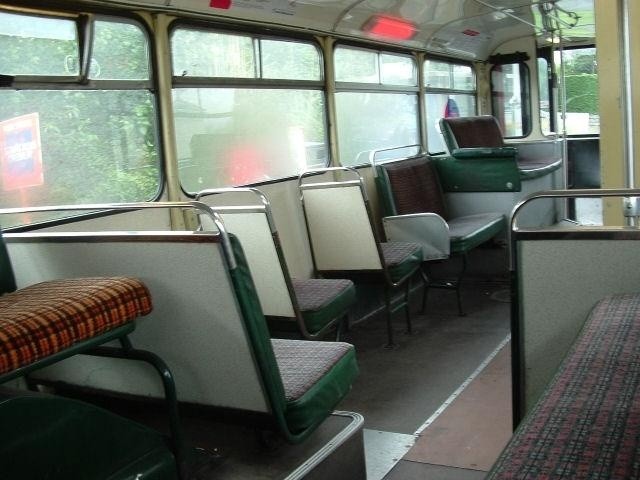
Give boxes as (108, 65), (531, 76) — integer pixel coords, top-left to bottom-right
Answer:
(196, 189), (358, 341)
(300, 169), (425, 347)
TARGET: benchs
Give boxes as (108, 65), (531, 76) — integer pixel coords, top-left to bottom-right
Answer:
(370, 111), (562, 317)
(1, 200), (361, 479)
(481, 291), (639, 478)
